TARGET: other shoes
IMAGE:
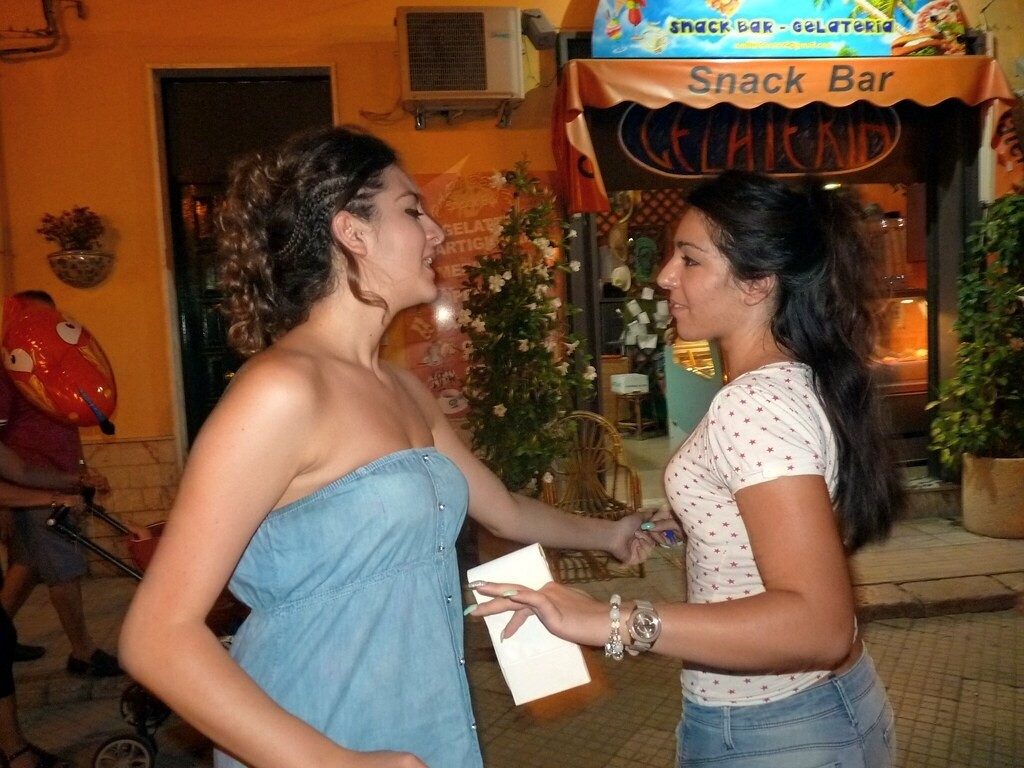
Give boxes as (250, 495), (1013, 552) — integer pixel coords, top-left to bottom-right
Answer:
(67, 647), (127, 678)
(11, 642), (45, 662)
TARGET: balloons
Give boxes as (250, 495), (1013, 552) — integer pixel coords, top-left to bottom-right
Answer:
(0, 294), (118, 440)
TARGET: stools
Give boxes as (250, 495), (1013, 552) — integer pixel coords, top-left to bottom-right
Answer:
(613, 394), (660, 441)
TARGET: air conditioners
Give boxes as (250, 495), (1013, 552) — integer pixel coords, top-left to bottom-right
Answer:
(396, 5), (527, 131)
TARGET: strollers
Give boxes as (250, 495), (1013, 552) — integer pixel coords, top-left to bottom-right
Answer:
(46, 482), (251, 768)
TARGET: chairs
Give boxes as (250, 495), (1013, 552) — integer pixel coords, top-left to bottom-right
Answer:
(538, 411), (645, 584)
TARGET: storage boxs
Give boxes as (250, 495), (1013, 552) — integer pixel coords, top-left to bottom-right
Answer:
(609, 373), (649, 394)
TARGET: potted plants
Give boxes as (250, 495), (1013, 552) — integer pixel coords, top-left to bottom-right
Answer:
(925, 184), (1024, 540)
(36, 206), (111, 250)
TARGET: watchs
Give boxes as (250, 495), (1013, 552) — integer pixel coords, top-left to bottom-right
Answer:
(624, 598), (664, 657)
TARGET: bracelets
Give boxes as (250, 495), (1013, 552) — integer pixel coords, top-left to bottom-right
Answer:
(605, 594), (623, 662)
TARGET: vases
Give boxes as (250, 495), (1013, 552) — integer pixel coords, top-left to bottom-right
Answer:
(48, 251), (114, 289)
(476, 520), (527, 564)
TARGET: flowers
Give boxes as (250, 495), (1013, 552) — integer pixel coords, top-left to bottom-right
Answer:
(456, 154), (597, 497)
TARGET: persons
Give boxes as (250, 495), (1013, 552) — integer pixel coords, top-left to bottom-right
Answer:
(463, 166), (908, 768)
(0, 284), (124, 768)
(113, 127), (656, 768)
